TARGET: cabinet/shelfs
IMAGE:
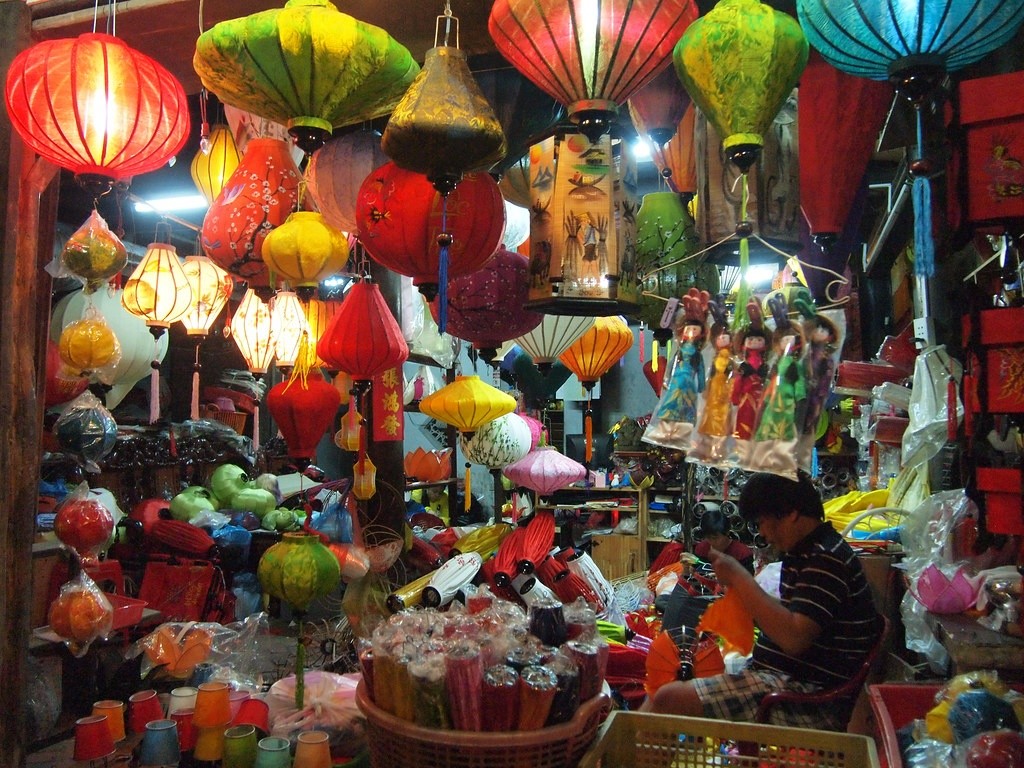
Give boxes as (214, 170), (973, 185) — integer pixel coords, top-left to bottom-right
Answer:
(535, 450), (856, 581)
(394, 352), (458, 586)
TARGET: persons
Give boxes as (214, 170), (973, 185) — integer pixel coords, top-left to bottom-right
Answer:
(638, 470), (879, 732)
(693, 511), (755, 576)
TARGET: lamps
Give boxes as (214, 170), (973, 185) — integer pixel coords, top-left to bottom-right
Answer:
(74, 680), (331, 768)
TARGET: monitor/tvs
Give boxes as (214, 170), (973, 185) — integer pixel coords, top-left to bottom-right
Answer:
(566, 434), (614, 472)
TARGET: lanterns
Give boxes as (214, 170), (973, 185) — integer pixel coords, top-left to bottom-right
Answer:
(5, 0), (1023, 699)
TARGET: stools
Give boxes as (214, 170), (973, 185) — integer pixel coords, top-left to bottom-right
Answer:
(80, 559), (130, 648)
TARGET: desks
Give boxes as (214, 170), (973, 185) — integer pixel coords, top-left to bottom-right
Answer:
(923, 610), (1024, 677)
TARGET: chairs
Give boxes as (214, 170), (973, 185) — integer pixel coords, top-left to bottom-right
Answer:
(683, 612), (892, 768)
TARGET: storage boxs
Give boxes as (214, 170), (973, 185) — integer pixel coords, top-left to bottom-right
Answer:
(101, 591), (149, 630)
(354, 675), (1024, 768)
(858, 555), (901, 618)
